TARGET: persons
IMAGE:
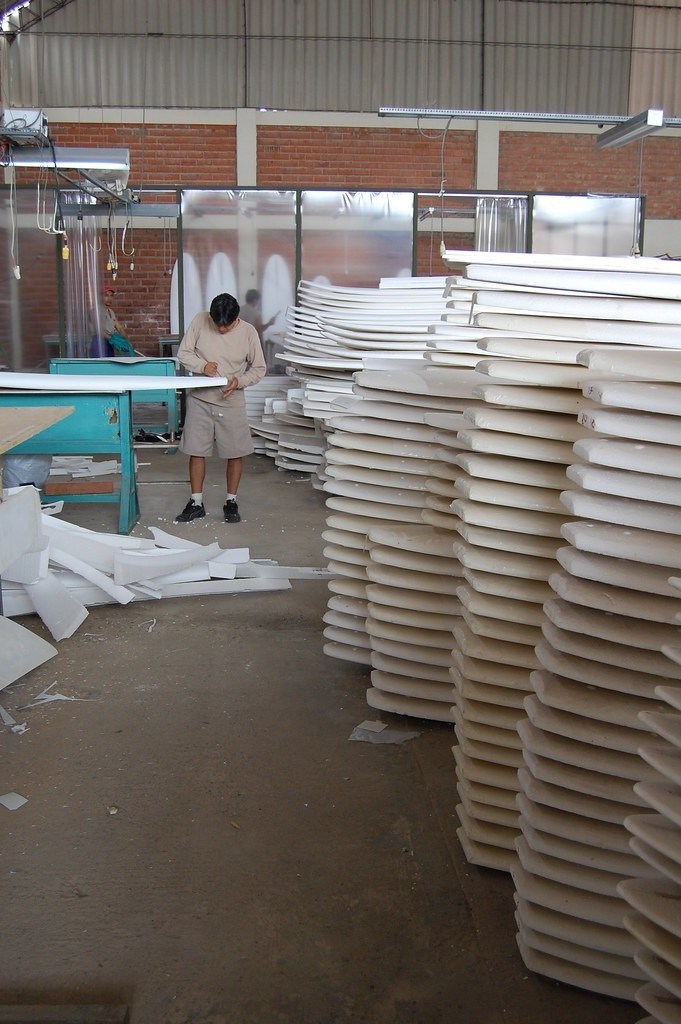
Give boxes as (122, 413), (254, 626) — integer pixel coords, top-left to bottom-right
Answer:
(238, 289), (275, 357)
(177, 293), (266, 523)
(87, 286), (130, 358)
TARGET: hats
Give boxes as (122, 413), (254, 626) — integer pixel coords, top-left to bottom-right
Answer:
(101, 286), (115, 294)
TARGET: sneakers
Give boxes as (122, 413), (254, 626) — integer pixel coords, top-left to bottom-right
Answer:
(223, 498), (240, 523)
(176, 498), (206, 522)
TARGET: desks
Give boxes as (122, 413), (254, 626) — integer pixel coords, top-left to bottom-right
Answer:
(157, 335), (180, 357)
(49, 359), (180, 454)
(0, 390), (141, 536)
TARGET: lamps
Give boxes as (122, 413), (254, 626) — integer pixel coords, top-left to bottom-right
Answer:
(596, 109), (663, 151)
(0, 147), (130, 170)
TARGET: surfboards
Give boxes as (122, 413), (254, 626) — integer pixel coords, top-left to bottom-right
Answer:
(0, 372), (229, 391)
(308, 275), (332, 302)
(261, 253), (294, 368)
(170, 253), (202, 357)
(243, 249), (681, 1024)
(205, 252), (237, 311)
